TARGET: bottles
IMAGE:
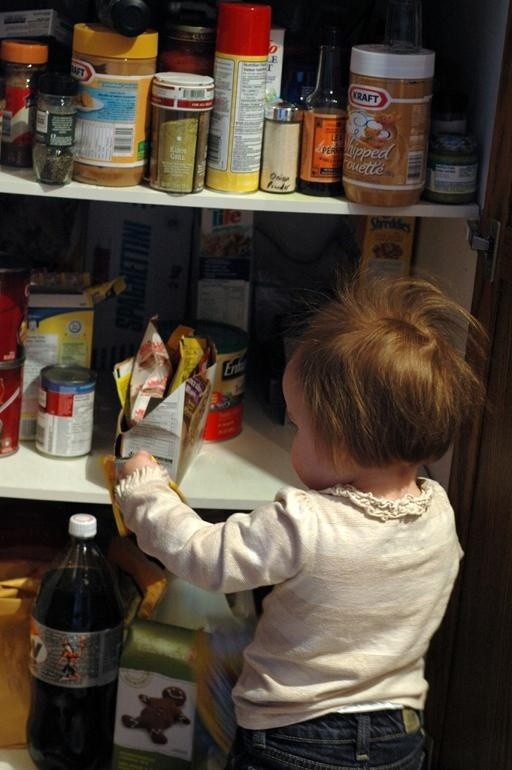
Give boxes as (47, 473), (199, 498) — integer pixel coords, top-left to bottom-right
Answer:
(31, 68), (76, 186)
(299, 29), (349, 190)
(425, 117), (481, 205)
(23, 513), (122, 769)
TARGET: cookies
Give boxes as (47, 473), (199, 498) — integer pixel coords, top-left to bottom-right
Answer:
(123, 686), (191, 746)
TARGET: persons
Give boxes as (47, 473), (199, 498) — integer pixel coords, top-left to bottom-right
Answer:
(111, 263), (493, 768)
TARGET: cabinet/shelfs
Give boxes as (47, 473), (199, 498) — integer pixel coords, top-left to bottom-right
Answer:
(1, 0), (511, 770)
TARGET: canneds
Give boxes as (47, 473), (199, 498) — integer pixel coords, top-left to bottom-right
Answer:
(342, 43), (435, 208)
(0, 359), (24, 458)
(0, 252), (31, 365)
(34, 363), (98, 458)
(190, 318), (249, 444)
(157, 24), (217, 72)
(421, 131), (480, 205)
(61, 23), (158, 186)
